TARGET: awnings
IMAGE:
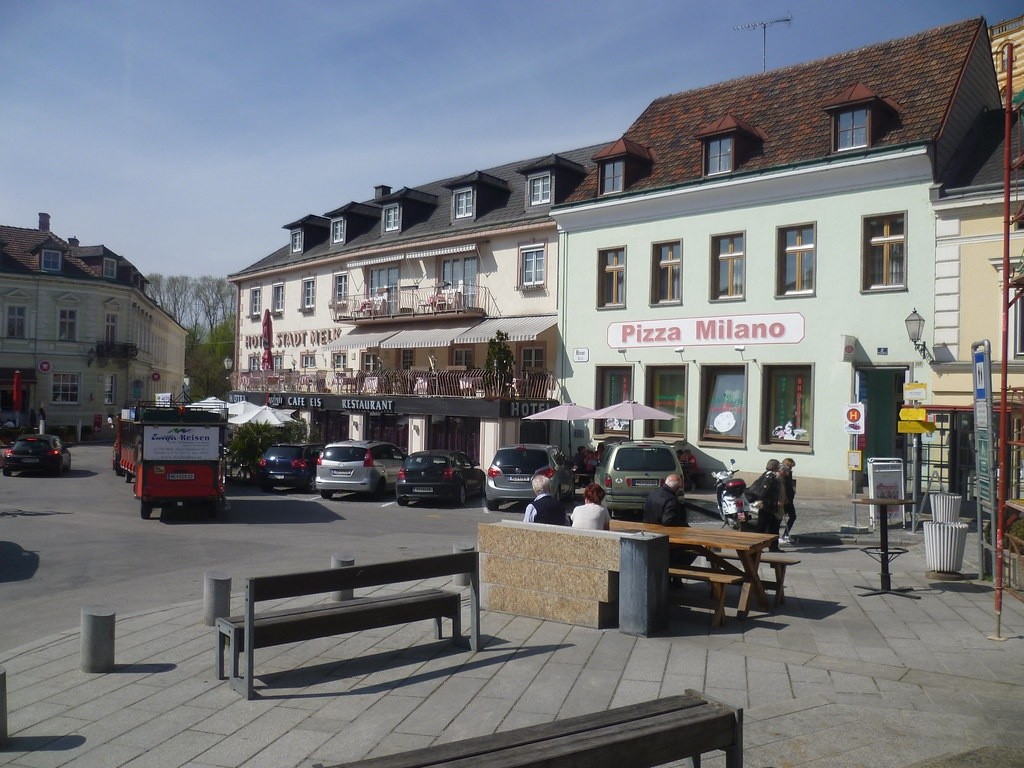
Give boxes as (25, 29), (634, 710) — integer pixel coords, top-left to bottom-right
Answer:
(346, 242), (479, 268)
(0, 367), (36, 385)
(322, 316), (560, 350)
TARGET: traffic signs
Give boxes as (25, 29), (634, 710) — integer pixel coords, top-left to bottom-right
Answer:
(898, 409), (937, 435)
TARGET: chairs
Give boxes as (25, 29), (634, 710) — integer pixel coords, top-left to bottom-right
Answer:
(417, 297), (432, 313)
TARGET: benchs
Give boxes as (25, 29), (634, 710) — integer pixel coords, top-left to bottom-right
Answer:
(215, 551), (479, 700)
(312, 690), (743, 768)
(695, 552), (801, 605)
(669, 564), (744, 629)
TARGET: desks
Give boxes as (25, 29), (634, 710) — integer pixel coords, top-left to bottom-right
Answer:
(609, 519), (780, 621)
(851, 498), (921, 599)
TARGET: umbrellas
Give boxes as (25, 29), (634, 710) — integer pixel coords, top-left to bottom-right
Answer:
(521, 398), (680, 498)
(12, 368), (23, 426)
(185, 395), (301, 427)
(261, 310), (273, 369)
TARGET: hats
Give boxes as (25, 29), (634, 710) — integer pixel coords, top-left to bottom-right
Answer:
(598, 443), (604, 448)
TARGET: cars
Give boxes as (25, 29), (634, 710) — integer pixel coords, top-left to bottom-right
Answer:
(395, 450), (486, 506)
(594, 439), (684, 517)
(3, 434), (71, 477)
(257, 442), (327, 492)
(0, 427), (36, 468)
(484, 442), (575, 511)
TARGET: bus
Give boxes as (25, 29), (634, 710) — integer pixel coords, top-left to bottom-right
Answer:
(107, 400), (229, 519)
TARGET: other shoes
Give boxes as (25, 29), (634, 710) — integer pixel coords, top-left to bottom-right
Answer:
(684, 486), (691, 491)
(669, 576), (684, 588)
(769, 548), (785, 553)
(783, 535), (796, 543)
(690, 482), (697, 491)
(778, 538), (787, 544)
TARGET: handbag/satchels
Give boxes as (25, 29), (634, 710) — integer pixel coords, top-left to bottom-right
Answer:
(745, 470), (773, 503)
(792, 479), (797, 494)
(773, 501), (784, 521)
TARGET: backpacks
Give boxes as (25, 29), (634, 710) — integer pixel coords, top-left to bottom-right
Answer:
(759, 472), (778, 505)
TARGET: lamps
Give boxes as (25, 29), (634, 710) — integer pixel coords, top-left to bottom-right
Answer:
(675, 347), (696, 363)
(735, 346), (756, 363)
(618, 347), (640, 364)
(905, 308), (926, 360)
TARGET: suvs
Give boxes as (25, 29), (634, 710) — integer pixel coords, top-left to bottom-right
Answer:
(314, 438), (407, 501)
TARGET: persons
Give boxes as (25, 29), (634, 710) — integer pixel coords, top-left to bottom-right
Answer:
(946, 419), (973, 494)
(107, 414), (115, 428)
(4, 407), (46, 429)
(522, 444), (796, 589)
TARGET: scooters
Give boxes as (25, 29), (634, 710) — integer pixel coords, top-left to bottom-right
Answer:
(711, 458), (752, 532)
